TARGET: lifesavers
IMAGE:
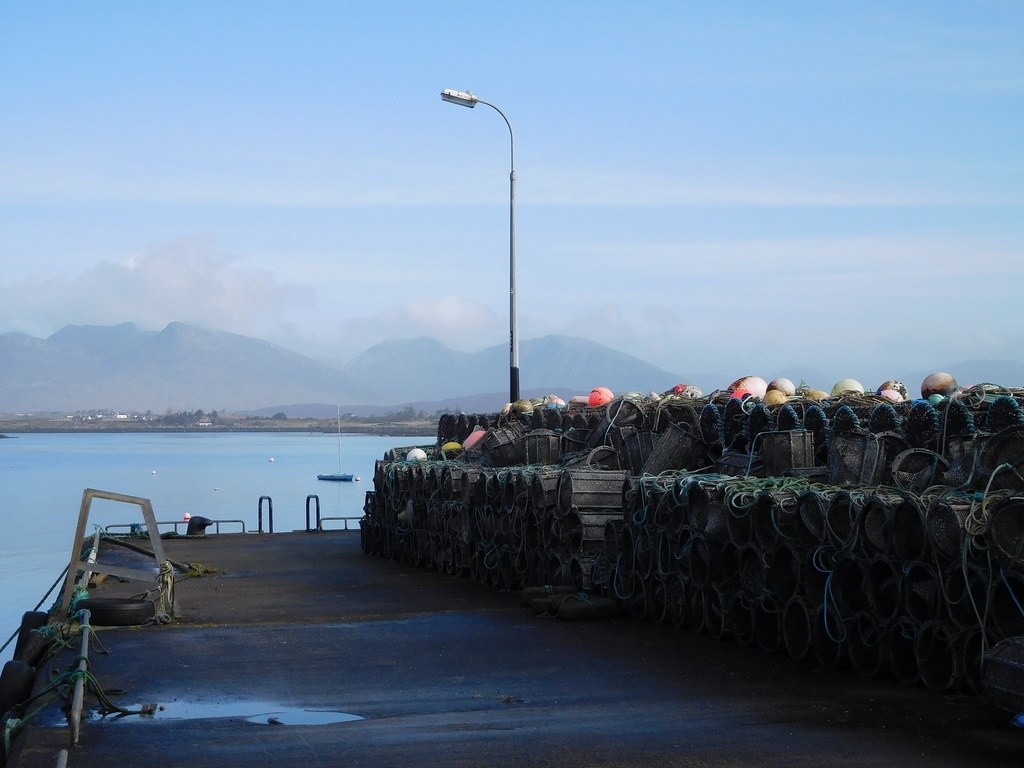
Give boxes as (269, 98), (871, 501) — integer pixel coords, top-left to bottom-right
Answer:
(76, 597), (157, 625)
(15, 629), (49, 664)
(12, 610), (49, 660)
(0, 660), (35, 717)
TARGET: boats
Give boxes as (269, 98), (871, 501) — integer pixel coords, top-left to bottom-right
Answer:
(317, 473), (354, 481)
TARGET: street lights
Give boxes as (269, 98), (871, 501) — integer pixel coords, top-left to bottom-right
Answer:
(440, 88), (520, 404)
